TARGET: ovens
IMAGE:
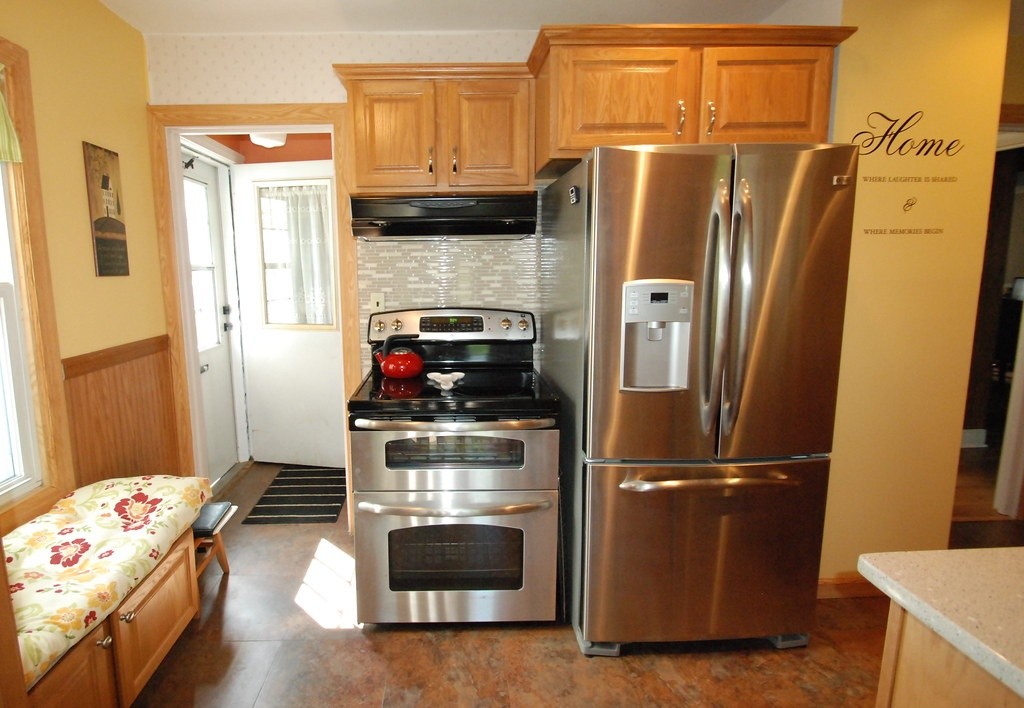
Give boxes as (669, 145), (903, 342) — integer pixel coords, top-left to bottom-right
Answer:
(348, 413), (560, 623)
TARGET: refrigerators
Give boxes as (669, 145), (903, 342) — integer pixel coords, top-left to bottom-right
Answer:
(536, 142), (860, 658)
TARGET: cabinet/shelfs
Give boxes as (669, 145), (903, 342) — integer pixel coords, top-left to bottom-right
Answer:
(330, 22), (860, 199)
(31, 527), (202, 708)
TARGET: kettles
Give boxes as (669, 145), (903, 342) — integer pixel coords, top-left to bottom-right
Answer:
(372, 334), (424, 380)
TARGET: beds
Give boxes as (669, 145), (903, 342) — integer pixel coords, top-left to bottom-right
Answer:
(0, 472), (213, 693)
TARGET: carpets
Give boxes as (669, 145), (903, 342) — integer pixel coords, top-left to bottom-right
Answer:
(241, 465), (346, 523)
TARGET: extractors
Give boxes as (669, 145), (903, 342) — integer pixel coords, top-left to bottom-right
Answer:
(351, 192), (539, 242)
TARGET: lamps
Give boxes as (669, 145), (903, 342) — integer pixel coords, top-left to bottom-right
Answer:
(250, 134), (288, 149)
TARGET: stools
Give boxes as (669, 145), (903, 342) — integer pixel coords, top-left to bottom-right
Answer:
(192, 501), (238, 581)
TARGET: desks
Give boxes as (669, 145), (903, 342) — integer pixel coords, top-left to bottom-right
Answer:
(857, 546), (1024, 701)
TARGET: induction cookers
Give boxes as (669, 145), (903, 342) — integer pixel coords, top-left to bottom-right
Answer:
(345, 306), (565, 415)
